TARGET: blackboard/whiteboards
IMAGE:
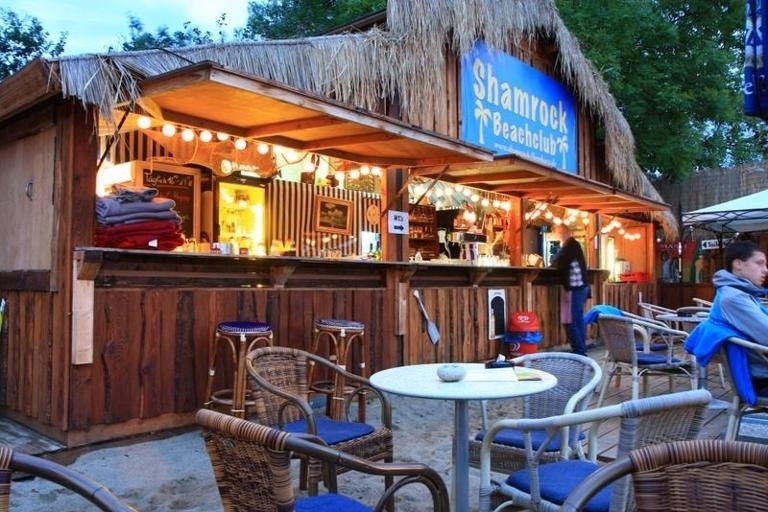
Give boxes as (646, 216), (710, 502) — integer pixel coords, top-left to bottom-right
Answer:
(131, 160), (201, 243)
(313, 195), (354, 237)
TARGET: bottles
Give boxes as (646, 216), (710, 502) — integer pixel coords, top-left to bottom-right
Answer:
(239, 236), (248, 254)
(368, 241), (380, 258)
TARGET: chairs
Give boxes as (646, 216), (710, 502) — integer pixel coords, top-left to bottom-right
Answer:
(455, 351), (604, 475)
(475, 385), (714, 511)
(242, 346), (396, 509)
(1, 444), (139, 512)
(595, 312), (701, 409)
(709, 326), (767, 441)
(596, 298), (767, 349)
(553, 440), (768, 511)
(196, 408), (455, 511)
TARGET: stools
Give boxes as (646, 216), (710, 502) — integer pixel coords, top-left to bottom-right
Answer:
(308, 318), (369, 422)
(202, 320), (276, 422)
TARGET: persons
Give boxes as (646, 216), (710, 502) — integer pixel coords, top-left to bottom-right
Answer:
(709, 238), (768, 399)
(550, 222), (592, 356)
(660, 250), (680, 282)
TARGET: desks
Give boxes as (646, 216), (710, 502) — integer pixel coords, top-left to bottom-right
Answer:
(368, 360), (561, 509)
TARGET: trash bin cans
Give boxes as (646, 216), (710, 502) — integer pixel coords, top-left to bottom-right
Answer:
(508, 312), (542, 359)
